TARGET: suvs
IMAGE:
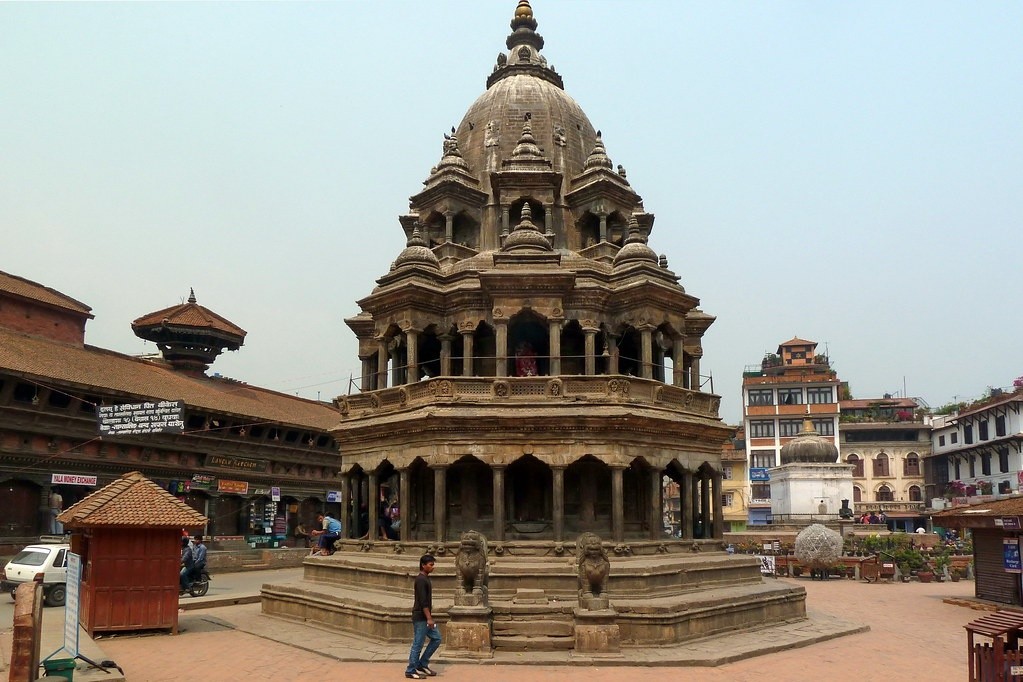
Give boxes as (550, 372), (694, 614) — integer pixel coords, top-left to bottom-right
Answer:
(0, 536), (70, 607)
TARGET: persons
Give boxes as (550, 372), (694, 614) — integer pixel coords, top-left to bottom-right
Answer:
(49, 487), (63, 535)
(945, 530), (957, 541)
(916, 525), (925, 534)
(312, 511), (341, 555)
(405, 555), (442, 679)
(180, 537), (195, 593)
(194, 535), (207, 584)
(860, 510), (887, 524)
(325, 500), (415, 541)
(295, 521), (314, 548)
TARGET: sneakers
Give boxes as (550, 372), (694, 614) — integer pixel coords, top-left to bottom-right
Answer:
(421, 666), (436, 676)
(405, 669), (426, 679)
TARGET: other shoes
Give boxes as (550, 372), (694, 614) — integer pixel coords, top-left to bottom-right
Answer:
(185, 587), (191, 593)
(329, 547), (337, 555)
(193, 581), (200, 584)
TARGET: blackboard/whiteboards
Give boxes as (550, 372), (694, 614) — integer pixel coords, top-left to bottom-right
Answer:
(64, 553), (82, 657)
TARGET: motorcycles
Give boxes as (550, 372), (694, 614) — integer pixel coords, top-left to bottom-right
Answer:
(179, 571), (211, 597)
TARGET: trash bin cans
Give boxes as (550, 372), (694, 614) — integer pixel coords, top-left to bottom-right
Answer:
(859, 552), (895, 578)
(963, 610), (1023, 682)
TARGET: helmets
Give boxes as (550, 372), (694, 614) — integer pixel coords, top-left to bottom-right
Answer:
(182, 536), (189, 547)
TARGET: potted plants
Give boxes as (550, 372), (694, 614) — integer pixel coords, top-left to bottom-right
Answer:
(842, 532), (974, 582)
